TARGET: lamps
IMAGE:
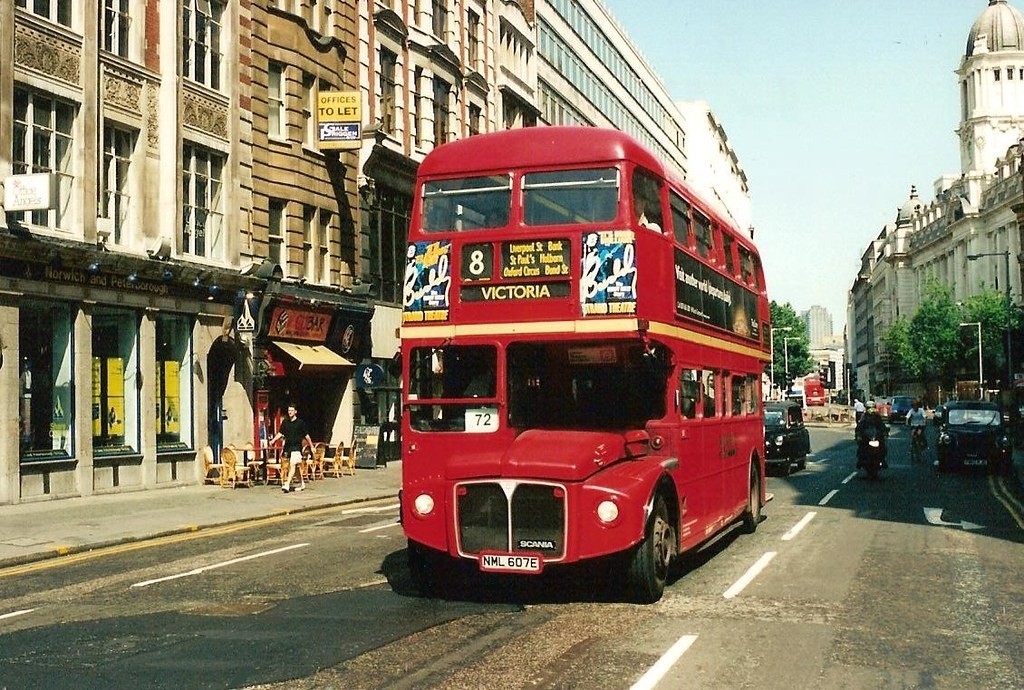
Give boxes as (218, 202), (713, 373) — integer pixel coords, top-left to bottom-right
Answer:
(239, 263), (261, 276)
(146, 238), (173, 258)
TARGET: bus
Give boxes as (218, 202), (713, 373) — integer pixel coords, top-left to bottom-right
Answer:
(805, 381), (825, 407)
(386, 126), (774, 603)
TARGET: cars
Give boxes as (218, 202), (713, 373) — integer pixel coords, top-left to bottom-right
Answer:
(932, 405), (946, 425)
(937, 401), (1013, 471)
(887, 395), (919, 425)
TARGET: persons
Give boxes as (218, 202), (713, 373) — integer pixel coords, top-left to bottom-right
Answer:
(854, 398), (928, 468)
(633, 191), (661, 234)
(268, 403), (316, 493)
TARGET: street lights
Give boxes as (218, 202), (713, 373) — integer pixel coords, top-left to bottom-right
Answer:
(959, 322), (985, 402)
(966, 250), (1013, 424)
(769, 327), (792, 401)
(782, 337), (801, 391)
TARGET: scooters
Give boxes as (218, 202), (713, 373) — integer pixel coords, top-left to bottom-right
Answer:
(859, 428), (889, 478)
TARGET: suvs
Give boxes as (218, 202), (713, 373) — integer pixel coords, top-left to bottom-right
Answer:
(761, 401), (812, 475)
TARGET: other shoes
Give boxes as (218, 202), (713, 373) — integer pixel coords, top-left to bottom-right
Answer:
(294, 483), (305, 491)
(282, 483), (289, 493)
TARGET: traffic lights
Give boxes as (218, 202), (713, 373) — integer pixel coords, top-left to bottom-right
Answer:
(848, 369), (858, 390)
(819, 367), (832, 389)
(786, 372), (792, 385)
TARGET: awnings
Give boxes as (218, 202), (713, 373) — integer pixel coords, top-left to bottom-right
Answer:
(272, 341), (356, 371)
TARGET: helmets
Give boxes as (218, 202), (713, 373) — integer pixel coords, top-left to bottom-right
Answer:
(865, 401), (876, 408)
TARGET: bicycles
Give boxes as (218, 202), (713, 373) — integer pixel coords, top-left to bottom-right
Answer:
(909, 428), (928, 461)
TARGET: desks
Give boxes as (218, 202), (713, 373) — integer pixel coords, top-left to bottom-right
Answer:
(236, 448), (284, 486)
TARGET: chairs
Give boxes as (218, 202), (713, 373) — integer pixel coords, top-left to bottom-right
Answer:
(205, 441), (354, 489)
